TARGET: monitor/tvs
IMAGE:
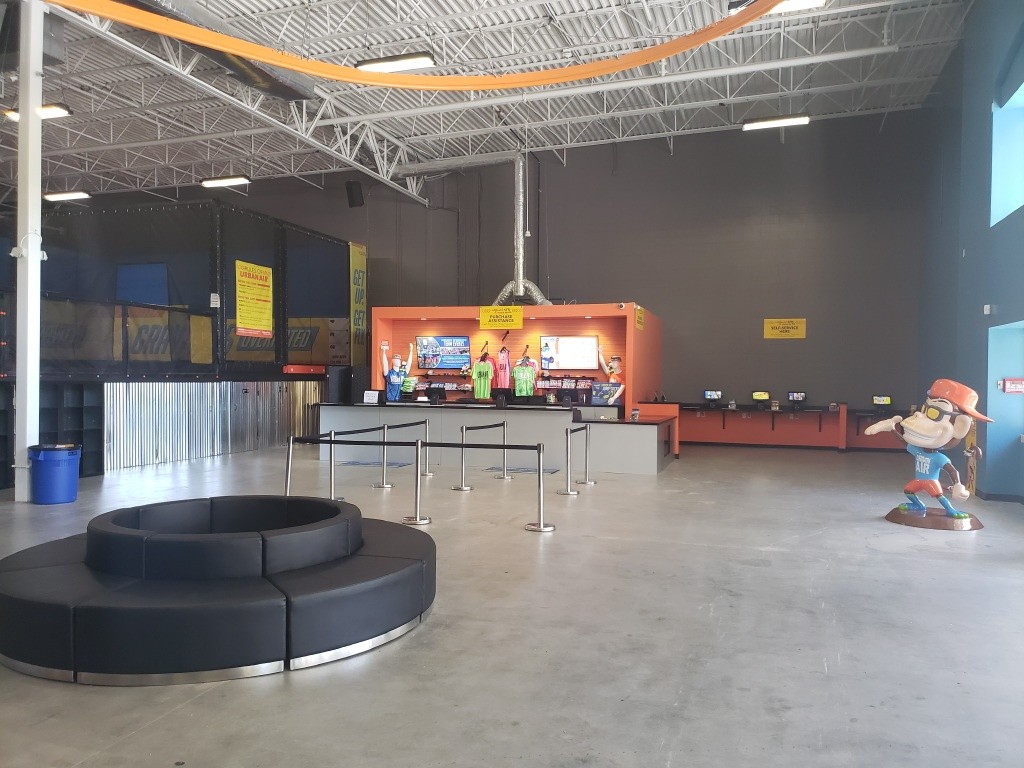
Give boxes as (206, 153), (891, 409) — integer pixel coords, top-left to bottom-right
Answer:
(704, 390), (722, 400)
(415, 335), (470, 368)
(540, 336), (598, 370)
(872, 395), (891, 405)
(788, 392), (806, 401)
(752, 391), (770, 400)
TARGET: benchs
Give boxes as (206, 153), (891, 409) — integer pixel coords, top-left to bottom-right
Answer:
(0, 493), (438, 689)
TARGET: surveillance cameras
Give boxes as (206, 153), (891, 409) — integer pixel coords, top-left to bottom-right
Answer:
(619, 302), (625, 309)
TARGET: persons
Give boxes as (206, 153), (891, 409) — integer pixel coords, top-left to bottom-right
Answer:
(382, 343), (413, 401)
(598, 345), (626, 405)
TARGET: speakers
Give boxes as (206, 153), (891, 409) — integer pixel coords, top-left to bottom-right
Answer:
(346, 181), (364, 208)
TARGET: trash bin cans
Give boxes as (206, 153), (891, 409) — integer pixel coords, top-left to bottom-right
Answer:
(29, 444), (81, 505)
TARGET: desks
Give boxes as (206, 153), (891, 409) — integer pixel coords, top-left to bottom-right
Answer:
(678, 400), (921, 456)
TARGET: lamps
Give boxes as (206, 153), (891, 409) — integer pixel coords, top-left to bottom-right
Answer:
(352, 19), (441, 75)
(197, 154), (253, 190)
(41, 151), (95, 203)
(1, 74), (75, 125)
(722, 91), (812, 133)
(726, 0), (829, 19)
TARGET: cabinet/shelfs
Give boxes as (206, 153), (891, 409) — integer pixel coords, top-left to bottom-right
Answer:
(411, 370), (476, 393)
(535, 374), (599, 393)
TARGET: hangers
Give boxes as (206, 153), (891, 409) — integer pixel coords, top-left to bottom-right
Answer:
(478, 351), (490, 363)
(519, 349), (531, 368)
(498, 340), (509, 354)
